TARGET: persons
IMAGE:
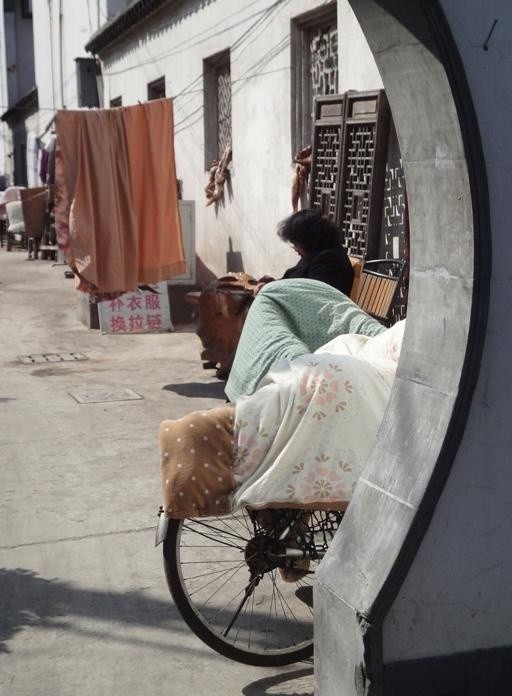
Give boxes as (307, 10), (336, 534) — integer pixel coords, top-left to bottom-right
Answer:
(252, 207), (355, 299)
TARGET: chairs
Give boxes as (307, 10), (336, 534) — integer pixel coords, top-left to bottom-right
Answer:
(5, 201), (26, 252)
(351, 255), (408, 327)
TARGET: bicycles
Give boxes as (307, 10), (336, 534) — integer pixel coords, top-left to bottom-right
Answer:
(154, 505), (345, 668)
